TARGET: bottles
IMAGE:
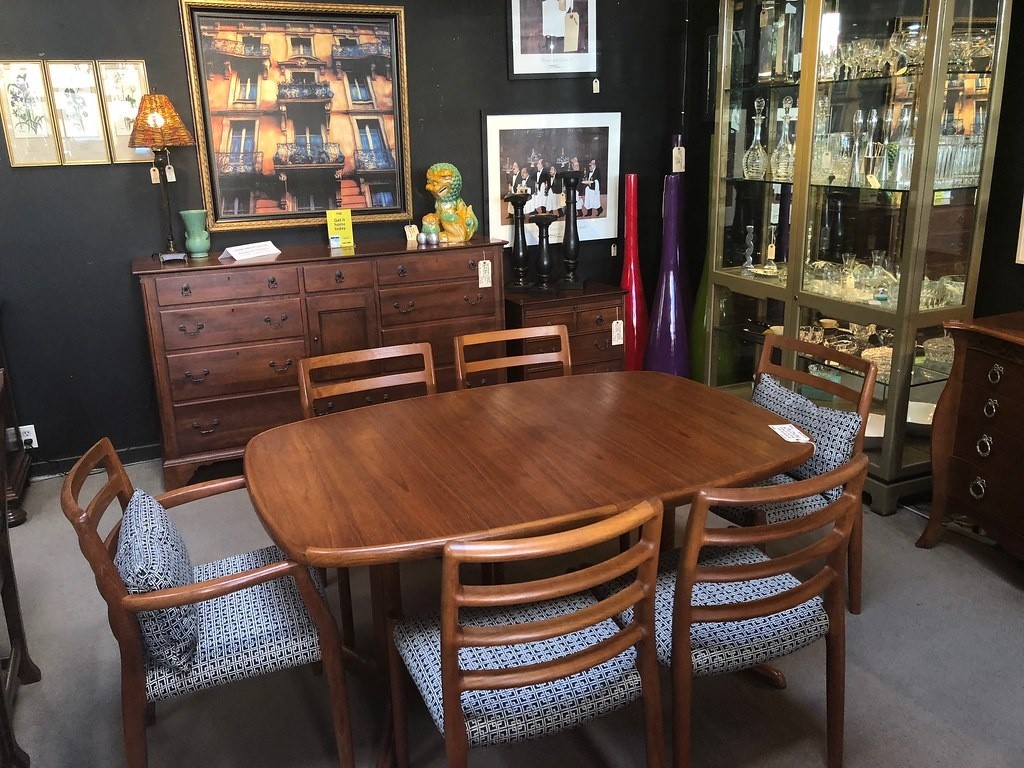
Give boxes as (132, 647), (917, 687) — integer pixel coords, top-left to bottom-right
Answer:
(771, 96), (795, 182)
(742, 96), (769, 181)
(810, 96), (833, 185)
(179, 210), (211, 258)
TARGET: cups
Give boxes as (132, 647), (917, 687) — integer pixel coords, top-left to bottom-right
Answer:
(899, 134), (964, 189)
(803, 250), (946, 311)
(819, 319), (836, 329)
(941, 275), (966, 307)
(770, 325), (783, 335)
(800, 326), (824, 345)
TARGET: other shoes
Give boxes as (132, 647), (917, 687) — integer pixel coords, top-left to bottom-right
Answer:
(507, 214), (513, 219)
(577, 213), (584, 217)
(585, 212), (592, 217)
(596, 208), (603, 216)
(529, 211), (539, 215)
(558, 212), (564, 218)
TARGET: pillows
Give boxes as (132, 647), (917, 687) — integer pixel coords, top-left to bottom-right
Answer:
(113, 487), (198, 673)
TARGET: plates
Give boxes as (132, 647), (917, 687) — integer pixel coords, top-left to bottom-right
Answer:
(748, 269), (781, 277)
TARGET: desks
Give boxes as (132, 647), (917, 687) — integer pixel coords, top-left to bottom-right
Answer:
(242, 367), (816, 768)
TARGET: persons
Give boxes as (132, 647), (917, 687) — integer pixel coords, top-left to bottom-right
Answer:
(506, 157), (603, 219)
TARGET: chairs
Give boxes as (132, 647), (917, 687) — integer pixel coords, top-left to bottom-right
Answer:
(296, 342), (437, 419)
(600, 452), (871, 768)
(708, 334), (878, 615)
(452, 323), (573, 390)
(60, 437), (355, 768)
(381, 496), (667, 768)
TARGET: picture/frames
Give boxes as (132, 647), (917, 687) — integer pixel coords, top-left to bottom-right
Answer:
(0, 59), (62, 168)
(44, 59), (112, 165)
(506, 0), (602, 80)
(96, 59), (163, 164)
(177, 0), (414, 232)
(479, 106), (626, 254)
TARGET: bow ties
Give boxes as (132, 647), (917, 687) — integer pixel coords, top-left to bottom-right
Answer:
(537, 171), (540, 172)
(550, 176), (554, 177)
(589, 170), (593, 173)
(523, 178), (526, 180)
(513, 174), (516, 175)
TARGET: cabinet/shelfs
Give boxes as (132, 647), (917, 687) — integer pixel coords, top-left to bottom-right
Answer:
(505, 277), (630, 381)
(130, 233), (509, 493)
(701, 0), (1013, 517)
(915, 310), (1024, 564)
(0, 368), (33, 529)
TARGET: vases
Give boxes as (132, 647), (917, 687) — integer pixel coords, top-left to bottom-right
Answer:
(178, 209), (211, 258)
(646, 174), (690, 378)
(691, 134), (742, 387)
(622, 174), (649, 370)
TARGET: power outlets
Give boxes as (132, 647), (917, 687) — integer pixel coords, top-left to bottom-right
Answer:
(6, 427), (19, 452)
(18, 424), (39, 449)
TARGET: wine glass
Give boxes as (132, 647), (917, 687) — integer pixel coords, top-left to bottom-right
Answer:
(818, 29), (929, 82)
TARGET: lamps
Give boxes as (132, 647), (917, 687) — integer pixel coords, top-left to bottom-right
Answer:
(127, 87), (197, 265)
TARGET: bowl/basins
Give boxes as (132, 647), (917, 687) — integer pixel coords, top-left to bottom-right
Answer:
(860, 347), (894, 371)
(824, 334), (859, 355)
(808, 363), (839, 380)
(923, 338), (955, 362)
